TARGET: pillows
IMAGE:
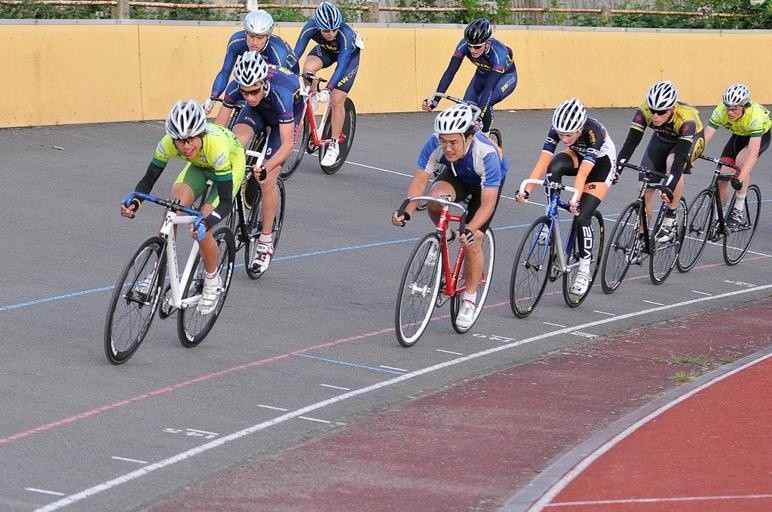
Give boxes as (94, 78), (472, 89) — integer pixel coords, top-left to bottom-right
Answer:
(464, 18), (492, 45)
(233, 51), (269, 87)
(723, 84), (749, 106)
(244, 10), (275, 36)
(552, 98), (587, 132)
(647, 81), (676, 111)
(314, 2), (342, 30)
(165, 100), (206, 140)
(433, 104), (472, 134)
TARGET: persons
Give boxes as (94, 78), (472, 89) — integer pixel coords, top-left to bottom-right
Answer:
(615, 79), (706, 264)
(421, 19), (518, 138)
(513, 97), (617, 299)
(704, 84), (772, 243)
(201, 9), (302, 130)
(214, 49), (309, 282)
(118, 98), (247, 317)
(288, 2), (365, 167)
(393, 101), (507, 333)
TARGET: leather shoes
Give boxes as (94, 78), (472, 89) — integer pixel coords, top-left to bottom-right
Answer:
(422, 99), (437, 109)
(252, 164), (266, 182)
(202, 98), (216, 115)
(515, 189), (529, 202)
(190, 220), (206, 241)
(392, 210), (411, 227)
(121, 197), (141, 218)
(569, 199), (580, 216)
(456, 228), (473, 247)
(732, 179), (743, 190)
(317, 90), (329, 103)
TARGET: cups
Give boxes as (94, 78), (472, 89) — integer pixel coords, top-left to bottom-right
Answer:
(321, 30), (336, 33)
(239, 89), (261, 95)
(467, 44), (482, 49)
(650, 108), (667, 115)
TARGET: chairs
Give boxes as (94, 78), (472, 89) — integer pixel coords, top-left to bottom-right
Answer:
(626, 238), (644, 265)
(570, 271), (589, 296)
(248, 239), (274, 274)
(425, 230), (455, 266)
(655, 217), (677, 243)
(321, 143), (340, 166)
(135, 272), (159, 297)
(455, 300), (476, 328)
(537, 224), (551, 246)
(197, 275), (222, 315)
(728, 208), (742, 229)
